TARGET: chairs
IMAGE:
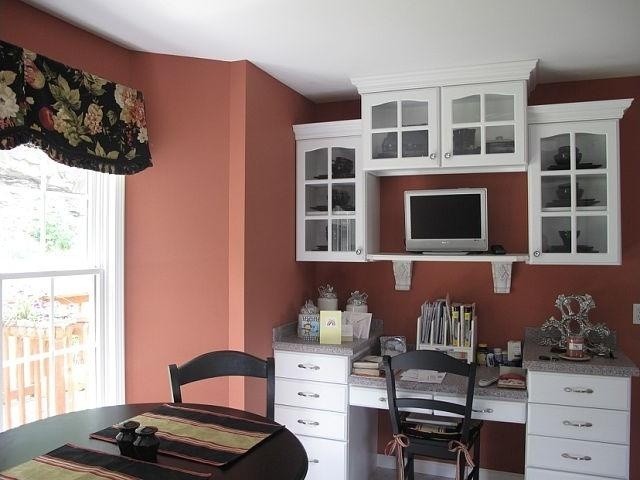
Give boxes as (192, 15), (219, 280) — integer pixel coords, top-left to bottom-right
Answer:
(378, 350), (483, 480)
(168, 350), (276, 422)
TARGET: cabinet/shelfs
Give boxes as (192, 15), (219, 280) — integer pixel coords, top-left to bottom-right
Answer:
(268, 319), (640, 480)
(291, 63), (633, 267)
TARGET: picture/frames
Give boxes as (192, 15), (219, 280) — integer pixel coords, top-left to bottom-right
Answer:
(378, 335), (408, 357)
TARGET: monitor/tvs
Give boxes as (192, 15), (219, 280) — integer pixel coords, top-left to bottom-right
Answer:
(404, 188), (488, 256)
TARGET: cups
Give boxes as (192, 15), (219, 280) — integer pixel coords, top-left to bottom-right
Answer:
(566, 336), (589, 356)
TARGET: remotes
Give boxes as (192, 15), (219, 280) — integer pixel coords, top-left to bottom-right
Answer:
(491, 245), (506, 254)
(478, 376), (499, 386)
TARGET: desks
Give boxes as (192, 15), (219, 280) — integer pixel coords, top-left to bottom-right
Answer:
(0, 401), (309, 479)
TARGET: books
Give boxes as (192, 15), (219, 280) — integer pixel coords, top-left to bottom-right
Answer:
(465, 303), (472, 347)
(352, 355), (383, 369)
(452, 302), (462, 345)
(497, 366), (526, 389)
(352, 369), (384, 376)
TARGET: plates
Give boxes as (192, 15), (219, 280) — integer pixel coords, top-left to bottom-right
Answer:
(558, 352), (591, 361)
